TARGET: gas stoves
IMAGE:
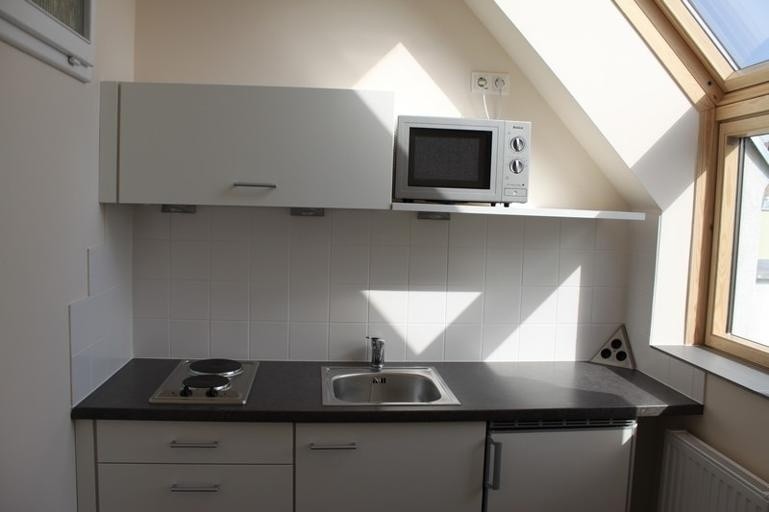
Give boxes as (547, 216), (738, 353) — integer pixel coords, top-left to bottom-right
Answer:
(147, 357), (261, 407)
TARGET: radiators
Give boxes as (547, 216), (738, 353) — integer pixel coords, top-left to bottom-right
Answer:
(656, 429), (768, 511)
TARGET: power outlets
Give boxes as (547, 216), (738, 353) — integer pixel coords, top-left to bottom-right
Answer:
(470, 71), (511, 96)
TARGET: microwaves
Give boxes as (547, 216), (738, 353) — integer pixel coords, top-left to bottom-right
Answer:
(392, 115), (533, 207)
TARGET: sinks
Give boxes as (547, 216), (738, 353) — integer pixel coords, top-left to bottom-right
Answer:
(321, 365), (461, 406)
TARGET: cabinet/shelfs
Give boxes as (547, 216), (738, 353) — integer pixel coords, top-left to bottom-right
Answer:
(74, 418), (297, 512)
(484, 424), (637, 512)
(293, 421), (484, 511)
(99, 81), (396, 210)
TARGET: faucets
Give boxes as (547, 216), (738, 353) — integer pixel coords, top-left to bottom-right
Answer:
(363, 336), (385, 370)
